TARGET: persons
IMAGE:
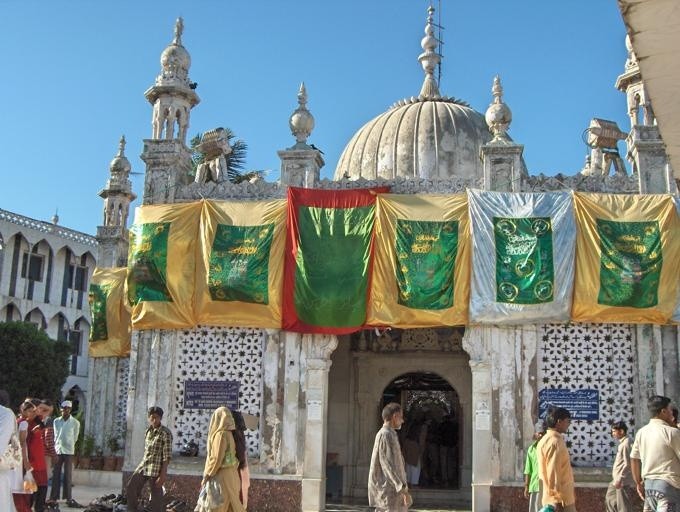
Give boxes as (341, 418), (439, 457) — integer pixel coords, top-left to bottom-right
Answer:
(0, 390), (59, 512)
(194, 406), (247, 512)
(537, 407), (577, 512)
(229, 410), (250, 510)
(605, 421), (634, 512)
(47, 400), (83, 508)
(395, 411), (459, 489)
(523, 432), (545, 512)
(368, 403), (413, 512)
(126, 406), (173, 512)
(630, 395), (680, 512)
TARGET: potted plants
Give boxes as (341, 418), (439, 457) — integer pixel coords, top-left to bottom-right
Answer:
(73, 435), (124, 471)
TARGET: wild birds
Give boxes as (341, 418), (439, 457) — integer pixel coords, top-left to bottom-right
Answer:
(309, 143), (325, 155)
(188, 82), (198, 93)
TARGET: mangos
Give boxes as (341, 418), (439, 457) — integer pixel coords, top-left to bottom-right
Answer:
(24, 481), (36, 492)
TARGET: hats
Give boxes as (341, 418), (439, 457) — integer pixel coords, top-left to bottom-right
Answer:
(62, 400), (72, 407)
